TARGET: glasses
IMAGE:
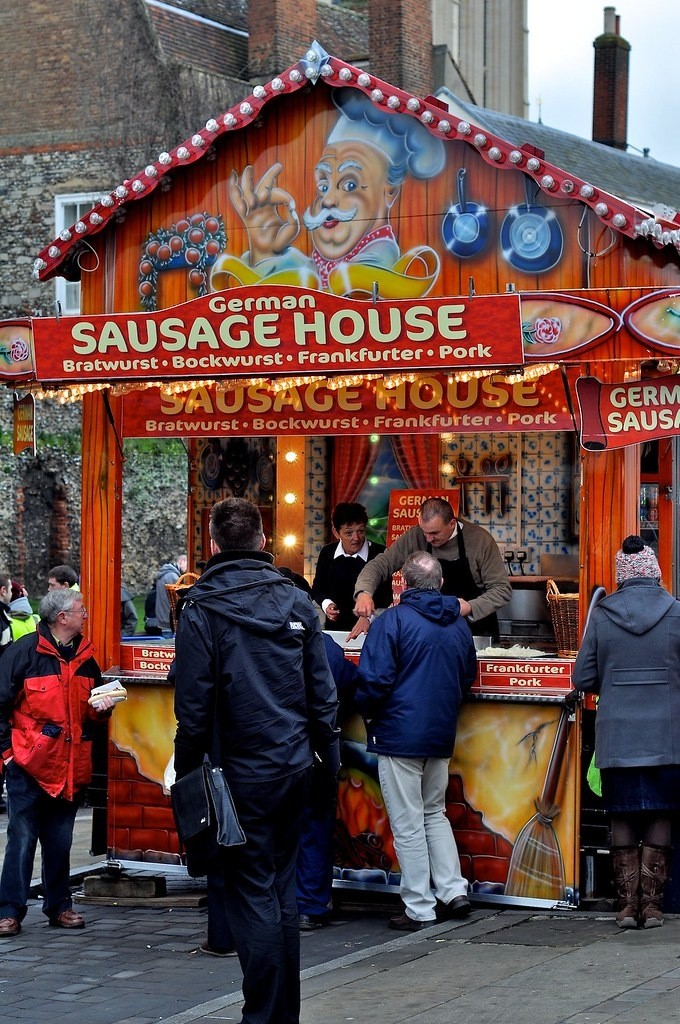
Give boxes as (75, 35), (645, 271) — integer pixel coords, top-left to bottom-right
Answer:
(57, 608), (86, 616)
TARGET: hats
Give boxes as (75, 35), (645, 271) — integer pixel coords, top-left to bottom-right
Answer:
(615, 535), (662, 584)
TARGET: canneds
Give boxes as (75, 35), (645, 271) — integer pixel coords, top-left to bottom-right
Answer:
(640, 484), (658, 521)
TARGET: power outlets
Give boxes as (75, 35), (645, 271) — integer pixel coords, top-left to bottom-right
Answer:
(497, 545), (531, 562)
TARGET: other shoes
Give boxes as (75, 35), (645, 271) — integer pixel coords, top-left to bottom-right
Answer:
(298, 909), (350, 929)
(200, 941), (238, 956)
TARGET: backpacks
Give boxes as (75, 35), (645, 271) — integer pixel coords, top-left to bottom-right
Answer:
(145, 572), (171, 619)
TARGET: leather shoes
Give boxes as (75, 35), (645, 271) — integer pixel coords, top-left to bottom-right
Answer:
(0, 917), (21, 935)
(49, 909), (85, 928)
(388, 913), (433, 932)
(435, 895), (471, 924)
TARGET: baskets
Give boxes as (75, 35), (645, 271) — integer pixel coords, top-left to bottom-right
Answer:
(165, 573), (201, 632)
(546, 579), (579, 659)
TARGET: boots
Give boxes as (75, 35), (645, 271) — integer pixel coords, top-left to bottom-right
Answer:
(638, 844), (667, 928)
(611, 844), (641, 927)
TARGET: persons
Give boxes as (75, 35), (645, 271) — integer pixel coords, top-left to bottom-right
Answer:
(174, 497), (341, 1024)
(569, 535), (680, 928)
(352, 497), (512, 645)
(172, 568), (360, 955)
(310, 503), (394, 643)
(353, 549), (476, 930)
(0, 555), (187, 810)
(0, 590), (114, 939)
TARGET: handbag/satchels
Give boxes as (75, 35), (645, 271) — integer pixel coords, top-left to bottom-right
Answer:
(170, 764), (247, 877)
(586, 696), (603, 797)
